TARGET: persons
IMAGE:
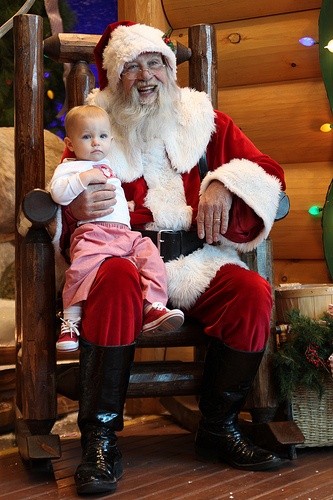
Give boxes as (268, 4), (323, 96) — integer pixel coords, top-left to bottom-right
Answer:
(50, 104), (185, 353)
(66, 18), (282, 495)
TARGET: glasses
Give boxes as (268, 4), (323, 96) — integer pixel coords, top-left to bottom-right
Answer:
(121, 64), (165, 80)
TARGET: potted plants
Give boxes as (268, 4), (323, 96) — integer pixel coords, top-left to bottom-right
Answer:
(265, 307), (333, 449)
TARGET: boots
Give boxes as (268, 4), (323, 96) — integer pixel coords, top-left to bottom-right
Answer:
(74, 336), (137, 493)
(193, 328), (275, 470)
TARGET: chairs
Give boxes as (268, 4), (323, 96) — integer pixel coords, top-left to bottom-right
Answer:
(12, 12), (307, 458)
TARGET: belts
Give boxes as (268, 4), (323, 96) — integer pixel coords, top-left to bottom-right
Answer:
(131, 228), (220, 264)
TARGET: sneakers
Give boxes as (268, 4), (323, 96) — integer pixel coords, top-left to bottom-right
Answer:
(56, 320), (79, 351)
(142, 302), (184, 336)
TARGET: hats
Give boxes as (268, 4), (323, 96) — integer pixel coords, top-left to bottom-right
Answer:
(86, 20), (177, 105)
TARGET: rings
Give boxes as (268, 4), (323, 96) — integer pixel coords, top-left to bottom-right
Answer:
(214, 218), (220, 220)
